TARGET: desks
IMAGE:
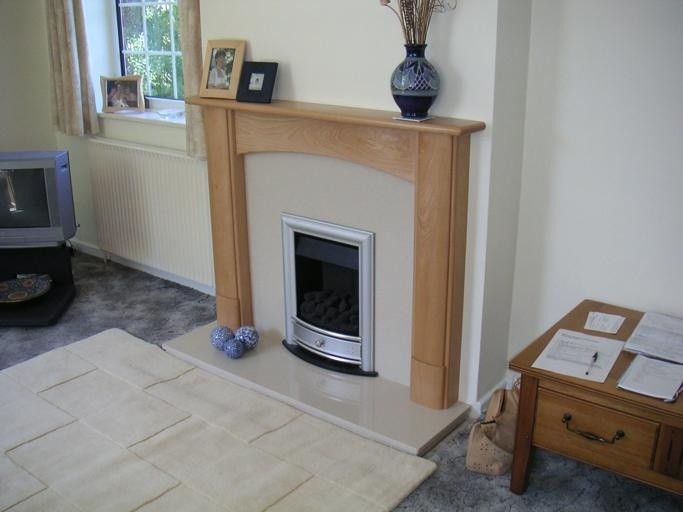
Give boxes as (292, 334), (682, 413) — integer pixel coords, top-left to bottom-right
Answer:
(509, 299), (683, 495)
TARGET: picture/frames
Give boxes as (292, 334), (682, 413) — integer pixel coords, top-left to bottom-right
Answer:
(236, 62), (277, 103)
(101, 76), (146, 113)
(198, 39), (246, 100)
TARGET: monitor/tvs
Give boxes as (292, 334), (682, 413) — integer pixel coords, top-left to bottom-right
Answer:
(0, 150), (77, 249)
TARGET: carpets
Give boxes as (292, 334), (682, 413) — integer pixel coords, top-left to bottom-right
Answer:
(0, 328), (438, 512)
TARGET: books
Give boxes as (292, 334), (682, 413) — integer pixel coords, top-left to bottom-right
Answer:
(616, 312), (683, 403)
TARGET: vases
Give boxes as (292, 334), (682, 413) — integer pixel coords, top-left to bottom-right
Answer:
(391, 44), (440, 123)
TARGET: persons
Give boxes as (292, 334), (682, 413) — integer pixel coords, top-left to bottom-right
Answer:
(208, 49), (229, 88)
(107, 80), (137, 106)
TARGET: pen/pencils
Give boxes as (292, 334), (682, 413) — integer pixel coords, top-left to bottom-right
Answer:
(586, 352), (598, 375)
